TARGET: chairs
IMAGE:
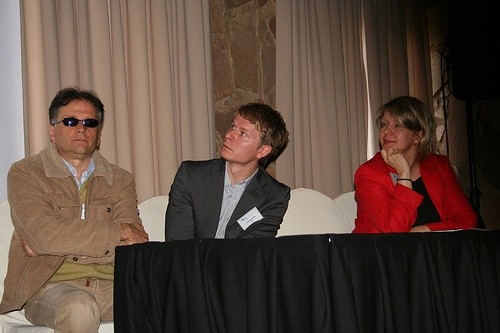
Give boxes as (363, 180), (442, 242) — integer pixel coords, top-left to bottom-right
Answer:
(0, 199), (114, 333)
(277, 189), (357, 238)
(137, 194), (170, 242)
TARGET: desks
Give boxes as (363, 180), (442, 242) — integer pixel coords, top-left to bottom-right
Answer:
(113, 230), (500, 333)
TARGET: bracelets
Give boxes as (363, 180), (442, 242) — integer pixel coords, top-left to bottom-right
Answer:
(395, 178), (412, 182)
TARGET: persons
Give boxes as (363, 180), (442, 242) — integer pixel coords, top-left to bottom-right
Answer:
(164, 101), (291, 241)
(0, 88), (149, 333)
(352, 96), (477, 233)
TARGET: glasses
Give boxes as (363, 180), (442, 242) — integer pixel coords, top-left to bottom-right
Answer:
(53, 118), (101, 128)
